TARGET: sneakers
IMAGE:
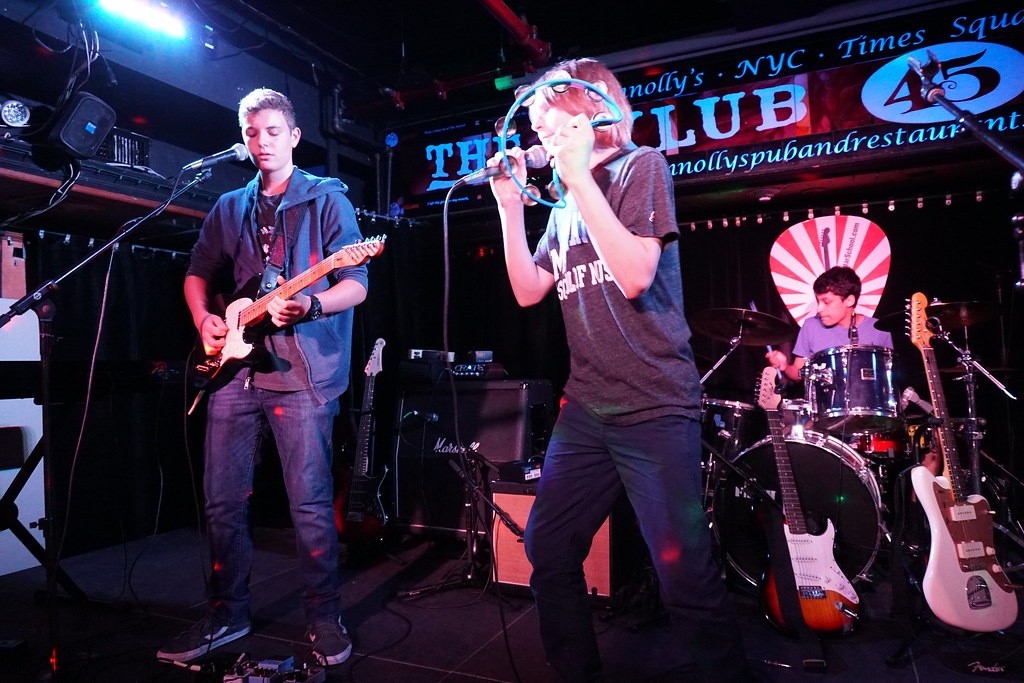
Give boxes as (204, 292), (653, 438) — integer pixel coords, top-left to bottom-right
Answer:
(156, 617), (253, 663)
(304, 619), (352, 666)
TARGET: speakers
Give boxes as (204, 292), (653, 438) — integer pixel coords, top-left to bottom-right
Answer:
(389, 379), (554, 543)
(489, 480), (634, 603)
(50, 91), (116, 160)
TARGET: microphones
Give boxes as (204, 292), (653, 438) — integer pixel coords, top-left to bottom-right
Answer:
(848, 312), (859, 338)
(460, 145), (550, 183)
(183, 144), (248, 172)
(901, 386), (920, 410)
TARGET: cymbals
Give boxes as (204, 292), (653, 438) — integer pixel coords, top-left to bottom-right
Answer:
(683, 304), (799, 348)
(937, 364), (1007, 374)
(876, 294), (982, 320)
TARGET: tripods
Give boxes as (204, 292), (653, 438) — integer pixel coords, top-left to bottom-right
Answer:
(398, 460), (522, 610)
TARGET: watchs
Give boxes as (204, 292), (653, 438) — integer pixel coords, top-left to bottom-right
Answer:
(307, 295), (323, 321)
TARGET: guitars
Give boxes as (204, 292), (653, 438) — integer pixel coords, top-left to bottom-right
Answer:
(753, 361), (873, 632)
(189, 233), (389, 389)
(903, 292), (1020, 632)
(330, 330), (390, 547)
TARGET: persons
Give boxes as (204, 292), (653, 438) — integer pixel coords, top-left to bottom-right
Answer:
(156, 89), (370, 667)
(765, 265), (893, 382)
(483, 56), (756, 683)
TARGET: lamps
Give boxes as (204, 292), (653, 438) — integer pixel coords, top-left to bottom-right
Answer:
(0, 100), (31, 128)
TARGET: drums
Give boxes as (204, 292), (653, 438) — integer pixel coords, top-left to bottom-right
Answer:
(847, 426), (903, 458)
(710, 431), (884, 591)
(802, 344), (902, 436)
(777, 397), (809, 439)
(700, 397), (766, 457)
